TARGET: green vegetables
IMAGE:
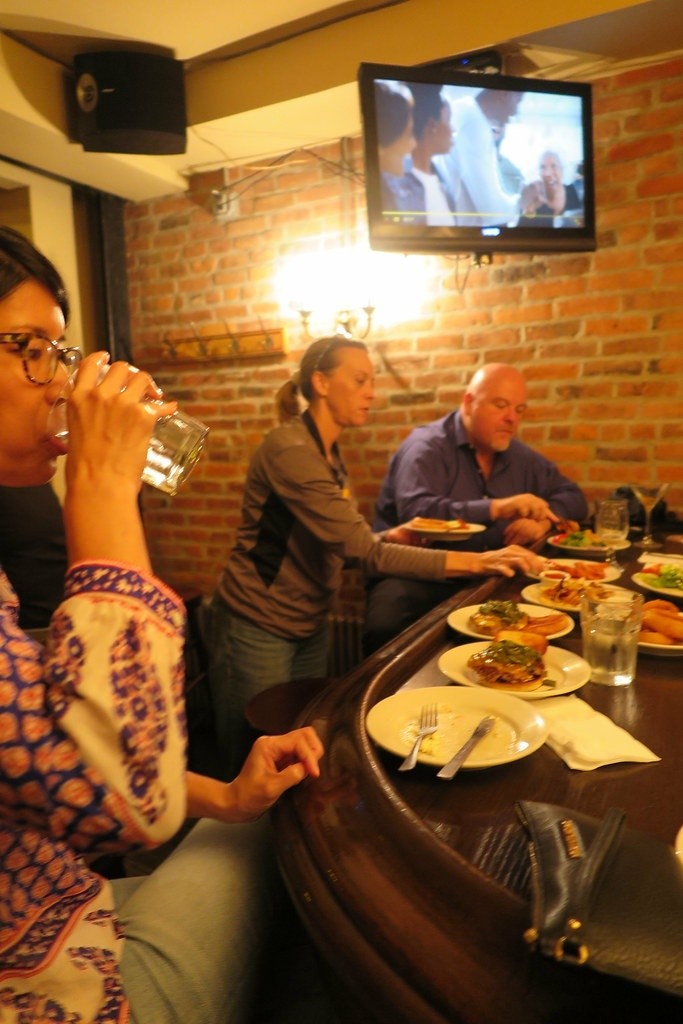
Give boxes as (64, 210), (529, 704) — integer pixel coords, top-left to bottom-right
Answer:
(644, 565), (683, 591)
(560, 532), (607, 547)
(478, 598), (526, 625)
(488, 640), (557, 687)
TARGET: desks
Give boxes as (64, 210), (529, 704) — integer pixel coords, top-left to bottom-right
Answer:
(273, 528), (683, 1024)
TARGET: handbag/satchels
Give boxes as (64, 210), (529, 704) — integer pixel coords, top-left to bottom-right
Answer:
(516, 796), (682, 1012)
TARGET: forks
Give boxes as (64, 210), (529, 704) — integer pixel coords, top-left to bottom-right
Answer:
(398, 703), (438, 771)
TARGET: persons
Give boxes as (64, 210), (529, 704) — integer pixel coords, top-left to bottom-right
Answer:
(211, 331), (547, 764)
(362, 361), (588, 656)
(384, 82), (460, 227)
(519, 150), (581, 229)
(432, 88), (524, 226)
(375, 78), (416, 226)
(0, 226), (325, 1024)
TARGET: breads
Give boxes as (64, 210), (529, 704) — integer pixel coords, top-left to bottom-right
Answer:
(518, 614), (567, 636)
(634, 598), (683, 647)
(493, 630), (548, 656)
(478, 675), (546, 692)
(411, 516), (450, 530)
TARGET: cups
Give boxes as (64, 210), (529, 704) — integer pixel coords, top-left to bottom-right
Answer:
(580, 587), (645, 684)
(44, 360), (209, 496)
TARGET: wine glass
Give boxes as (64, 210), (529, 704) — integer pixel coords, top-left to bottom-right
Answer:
(596, 499), (628, 573)
(629, 456), (672, 549)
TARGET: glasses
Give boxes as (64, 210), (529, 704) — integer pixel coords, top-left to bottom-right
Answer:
(0, 332), (82, 384)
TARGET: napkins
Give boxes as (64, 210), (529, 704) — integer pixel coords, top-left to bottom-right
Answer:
(637, 550), (683, 567)
(528, 694), (663, 770)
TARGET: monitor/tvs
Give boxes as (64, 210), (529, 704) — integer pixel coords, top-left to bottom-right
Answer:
(356, 62), (596, 252)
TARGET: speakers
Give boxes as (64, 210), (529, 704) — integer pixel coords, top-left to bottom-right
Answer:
(67, 49), (188, 155)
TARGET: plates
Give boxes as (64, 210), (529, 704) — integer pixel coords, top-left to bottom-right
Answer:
(525, 558), (621, 582)
(638, 611), (683, 656)
(521, 581), (645, 616)
(631, 571), (683, 598)
(547, 535), (631, 557)
(438, 641), (592, 700)
(447, 603), (575, 639)
(365, 686), (549, 769)
(400, 520), (486, 540)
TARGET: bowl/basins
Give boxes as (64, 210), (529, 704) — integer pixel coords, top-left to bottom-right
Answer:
(538, 570), (571, 583)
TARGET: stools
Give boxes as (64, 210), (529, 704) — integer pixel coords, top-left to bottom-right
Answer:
(244, 674), (325, 742)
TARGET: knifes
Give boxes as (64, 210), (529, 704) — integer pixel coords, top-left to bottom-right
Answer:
(436, 715), (495, 780)
